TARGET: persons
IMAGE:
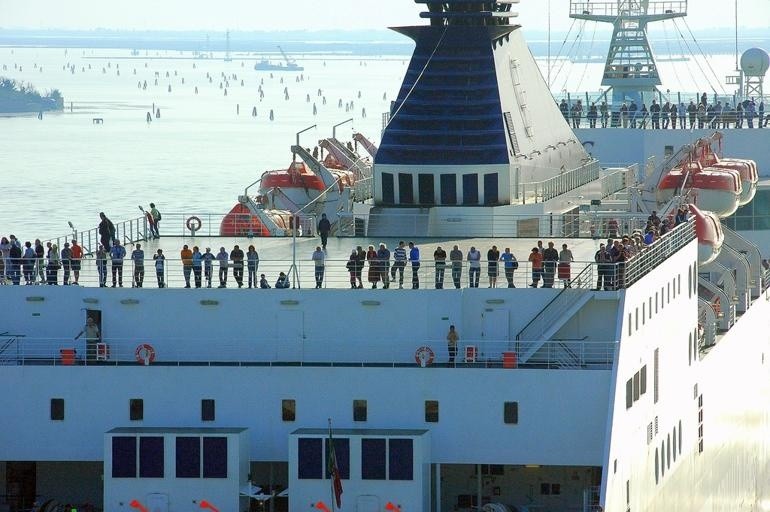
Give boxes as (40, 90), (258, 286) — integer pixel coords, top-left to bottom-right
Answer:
(356, 246), (365, 289)
(446, 325), (460, 367)
(311, 247), (325, 288)
(181, 243), (259, 288)
(434, 246), (446, 289)
(450, 245), (462, 288)
(467, 247), (481, 287)
(260, 274), (272, 289)
(275, 272), (290, 288)
(487, 246), (499, 288)
(528, 239), (573, 290)
(377, 243), (390, 289)
(346, 250), (357, 289)
(367, 245), (380, 289)
(96, 203), (165, 288)
(389, 241), (408, 289)
(499, 248), (518, 288)
(316, 213), (331, 250)
(408, 242), (420, 290)
(556, 93), (770, 130)
(74, 314), (100, 365)
(594, 208), (689, 290)
(0, 234), (83, 286)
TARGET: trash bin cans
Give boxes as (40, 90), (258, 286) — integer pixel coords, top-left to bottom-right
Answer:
(60, 348), (77, 366)
(501, 351), (517, 369)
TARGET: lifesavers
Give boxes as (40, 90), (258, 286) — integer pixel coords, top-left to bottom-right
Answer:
(134, 345), (155, 364)
(187, 217), (201, 231)
(415, 347), (433, 365)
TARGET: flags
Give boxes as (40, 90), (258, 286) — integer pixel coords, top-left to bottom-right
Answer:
(327, 428), (343, 509)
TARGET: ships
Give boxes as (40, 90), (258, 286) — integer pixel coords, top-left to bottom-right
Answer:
(0, 0), (770, 512)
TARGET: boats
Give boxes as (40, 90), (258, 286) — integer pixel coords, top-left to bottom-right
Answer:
(255, 45), (304, 71)
(570, 55), (690, 66)
